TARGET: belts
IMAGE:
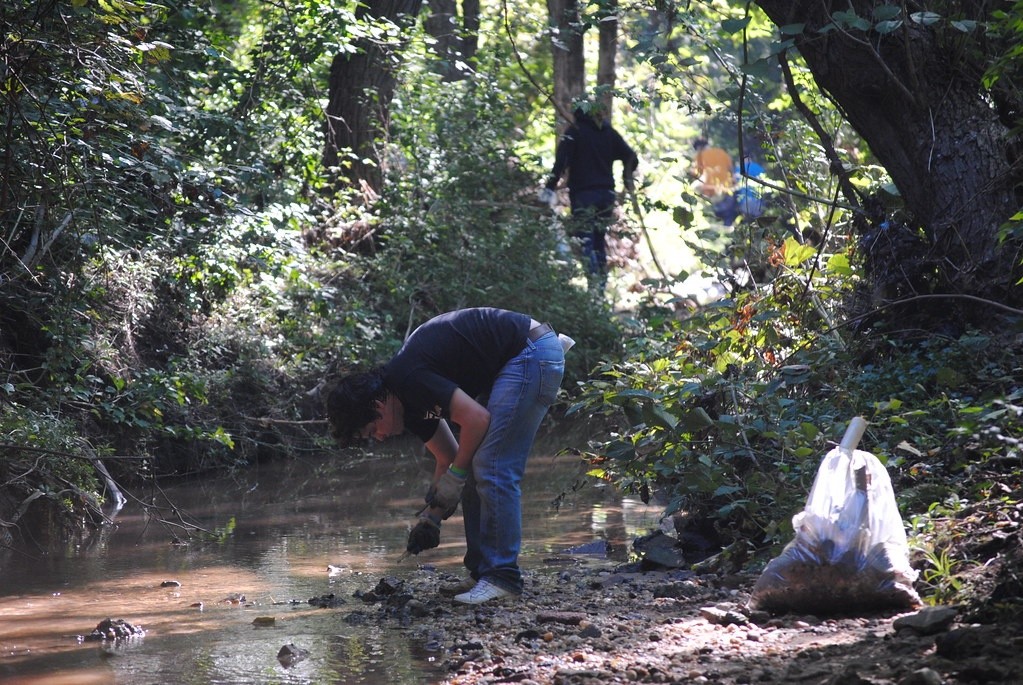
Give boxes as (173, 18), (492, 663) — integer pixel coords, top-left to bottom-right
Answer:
(522, 321), (552, 348)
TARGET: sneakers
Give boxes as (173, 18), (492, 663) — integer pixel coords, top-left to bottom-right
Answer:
(453, 578), (519, 604)
(439, 577), (477, 594)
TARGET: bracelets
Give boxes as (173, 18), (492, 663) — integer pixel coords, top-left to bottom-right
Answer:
(448, 463), (467, 477)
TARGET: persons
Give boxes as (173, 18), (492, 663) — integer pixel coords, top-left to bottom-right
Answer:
(544, 100), (639, 298)
(327, 304), (566, 607)
(733, 150), (768, 219)
(693, 140), (732, 197)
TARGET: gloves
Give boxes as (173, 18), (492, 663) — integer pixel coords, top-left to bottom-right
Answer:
(425, 471), (466, 520)
